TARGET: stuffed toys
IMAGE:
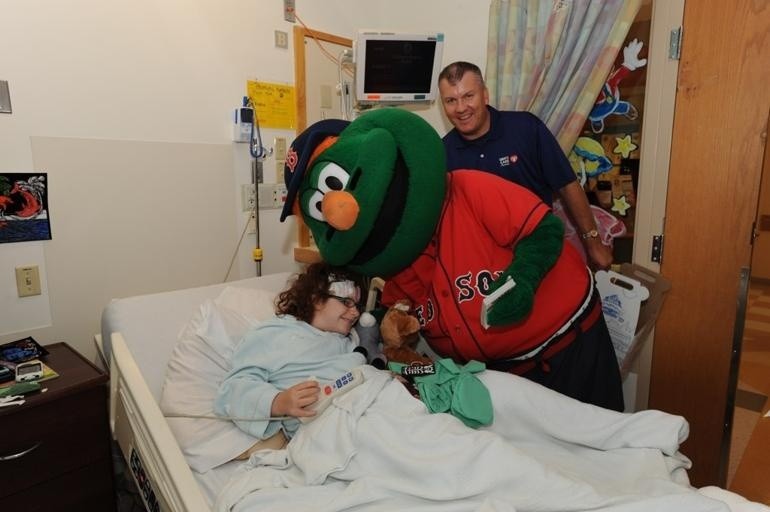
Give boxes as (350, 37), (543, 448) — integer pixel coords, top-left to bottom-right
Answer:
(278, 108), (626, 413)
(357, 275), (436, 401)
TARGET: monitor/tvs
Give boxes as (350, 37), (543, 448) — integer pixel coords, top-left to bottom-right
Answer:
(354, 31), (443, 101)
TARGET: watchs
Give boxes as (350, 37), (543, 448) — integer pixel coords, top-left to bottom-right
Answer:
(576, 228), (599, 244)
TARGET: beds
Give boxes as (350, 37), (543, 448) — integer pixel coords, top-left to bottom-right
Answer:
(95, 273), (769, 512)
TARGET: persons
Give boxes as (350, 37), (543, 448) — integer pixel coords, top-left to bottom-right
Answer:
(437, 61), (613, 272)
(212, 262), (615, 510)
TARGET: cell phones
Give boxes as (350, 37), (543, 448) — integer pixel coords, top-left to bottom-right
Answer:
(15, 361), (43, 382)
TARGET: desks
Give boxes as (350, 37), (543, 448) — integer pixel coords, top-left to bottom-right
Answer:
(1, 342), (109, 413)
